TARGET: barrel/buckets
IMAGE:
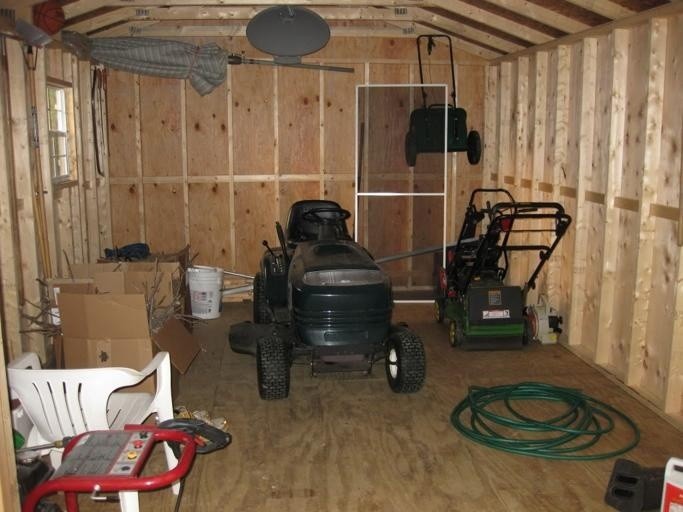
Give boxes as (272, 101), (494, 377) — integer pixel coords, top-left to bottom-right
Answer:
(188, 268), (224, 320)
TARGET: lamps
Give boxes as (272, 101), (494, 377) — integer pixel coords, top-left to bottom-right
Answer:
(245, 3), (330, 64)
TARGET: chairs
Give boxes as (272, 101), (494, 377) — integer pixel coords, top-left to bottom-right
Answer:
(6, 351), (182, 512)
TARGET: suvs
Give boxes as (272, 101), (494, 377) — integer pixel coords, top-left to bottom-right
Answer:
(228, 199), (425, 397)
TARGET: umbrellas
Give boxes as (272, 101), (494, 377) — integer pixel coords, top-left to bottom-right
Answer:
(65, 34), (355, 98)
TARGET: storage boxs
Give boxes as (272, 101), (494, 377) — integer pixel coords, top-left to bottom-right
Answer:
(71, 264), (125, 295)
(125, 262), (185, 322)
(48, 279), (94, 369)
(58, 272), (201, 402)
